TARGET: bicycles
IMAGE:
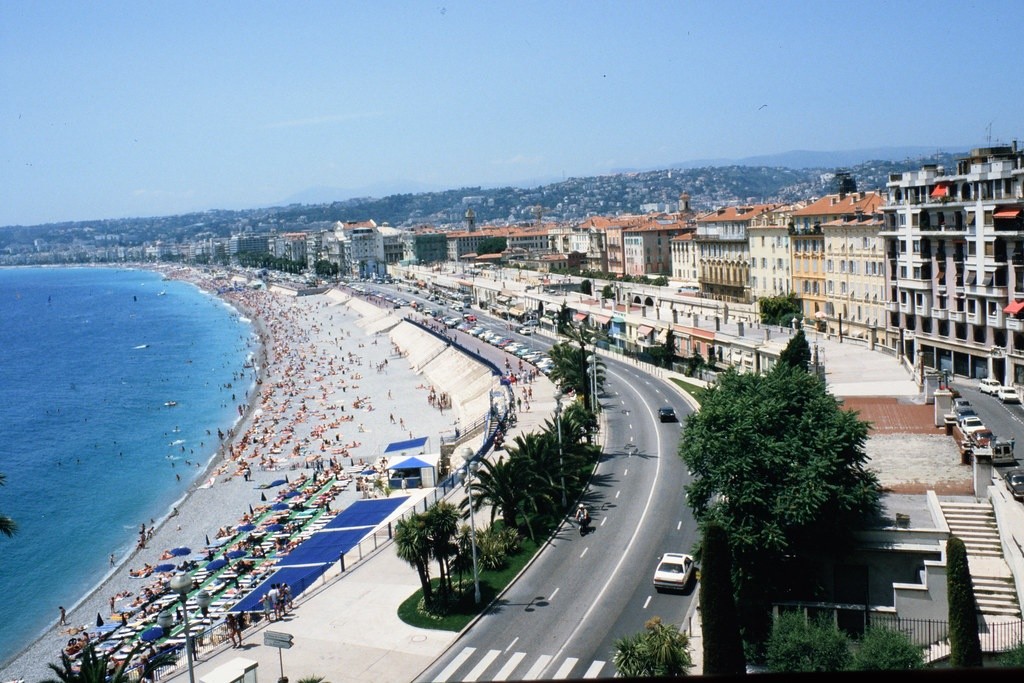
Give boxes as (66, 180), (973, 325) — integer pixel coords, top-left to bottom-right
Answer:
(576, 517), (589, 536)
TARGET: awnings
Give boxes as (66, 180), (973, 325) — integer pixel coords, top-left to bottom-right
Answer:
(954, 270), (976, 283)
(1003, 301), (1024, 313)
(931, 182), (952, 197)
(965, 213), (975, 224)
(936, 270), (945, 278)
(992, 208), (1024, 219)
(982, 273), (993, 285)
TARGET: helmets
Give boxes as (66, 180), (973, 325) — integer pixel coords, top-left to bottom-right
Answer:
(579, 504), (583, 508)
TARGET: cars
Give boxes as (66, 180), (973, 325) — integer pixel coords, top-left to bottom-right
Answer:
(1003, 469), (1024, 502)
(653, 552), (695, 593)
(940, 385), (1015, 466)
(233, 268), (568, 386)
(997, 386), (1020, 404)
(657, 406), (675, 423)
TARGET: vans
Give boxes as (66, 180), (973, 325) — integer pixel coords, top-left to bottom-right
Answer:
(978, 378), (1002, 396)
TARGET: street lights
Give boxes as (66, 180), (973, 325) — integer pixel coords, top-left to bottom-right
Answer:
(586, 354), (598, 446)
(156, 570), (212, 683)
(553, 312), (559, 345)
(552, 389), (568, 508)
(590, 336), (598, 409)
(455, 446), (481, 604)
(505, 301), (511, 338)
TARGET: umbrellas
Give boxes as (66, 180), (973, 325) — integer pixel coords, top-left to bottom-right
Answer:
(154, 564), (175, 573)
(271, 480), (286, 486)
(171, 547), (192, 564)
(271, 503), (289, 510)
(266, 524), (283, 532)
(286, 491), (301, 498)
(141, 627), (164, 641)
(237, 525), (256, 531)
(204, 559), (227, 571)
(226, 550), (247, 558)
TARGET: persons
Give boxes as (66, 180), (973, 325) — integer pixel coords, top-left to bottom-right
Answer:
(54, 264), (484, 683)
(504, 357), (539, 385)
(491, 386), (533, 450)
(575, 504), (590, 533)
